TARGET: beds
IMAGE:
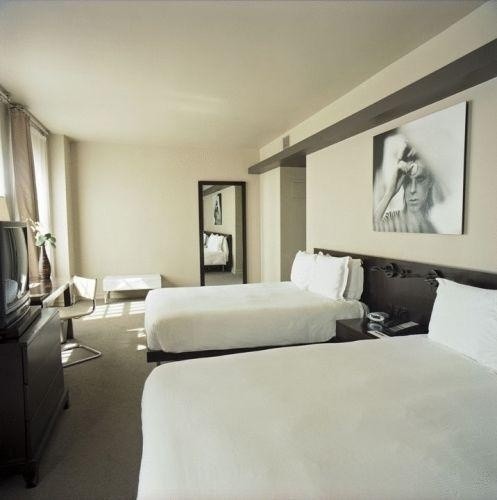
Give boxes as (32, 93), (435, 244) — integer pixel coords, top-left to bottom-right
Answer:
(144, 281), (369, 366)
(139, 334), (497, 500)
(203, 231), (233, 272)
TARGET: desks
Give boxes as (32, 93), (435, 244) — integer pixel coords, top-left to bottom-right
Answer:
(28, 280), (75, 339)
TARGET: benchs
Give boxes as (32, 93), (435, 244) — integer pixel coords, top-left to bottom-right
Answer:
(102, 274), (161, 304)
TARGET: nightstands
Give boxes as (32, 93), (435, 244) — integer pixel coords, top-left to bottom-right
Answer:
(334, 317), (428, 341)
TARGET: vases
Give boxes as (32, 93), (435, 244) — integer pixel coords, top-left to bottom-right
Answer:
(38, 244), (51, 279)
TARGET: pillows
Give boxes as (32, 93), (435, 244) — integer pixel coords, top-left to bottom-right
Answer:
(429, 279), (497, 371)
(291, 249), (365, 303)
(203, 233), (224, 251)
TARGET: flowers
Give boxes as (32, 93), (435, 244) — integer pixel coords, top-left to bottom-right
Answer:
(26, 217), (57, 249)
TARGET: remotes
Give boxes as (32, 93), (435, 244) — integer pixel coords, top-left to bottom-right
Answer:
(383, 321), (420, 336)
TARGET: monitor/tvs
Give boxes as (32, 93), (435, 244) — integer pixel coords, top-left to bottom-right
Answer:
(0, 221), (31, 330)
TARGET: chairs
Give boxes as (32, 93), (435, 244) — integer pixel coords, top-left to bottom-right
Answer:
(48, 275), (102, 368)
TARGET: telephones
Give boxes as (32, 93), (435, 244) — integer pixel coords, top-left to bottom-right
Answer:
(382, 319), (425, 336)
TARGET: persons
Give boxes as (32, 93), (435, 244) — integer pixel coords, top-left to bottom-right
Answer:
(373, 140), (444, 234)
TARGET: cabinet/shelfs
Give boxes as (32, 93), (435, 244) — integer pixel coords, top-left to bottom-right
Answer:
(0, 307), (71, 489)
